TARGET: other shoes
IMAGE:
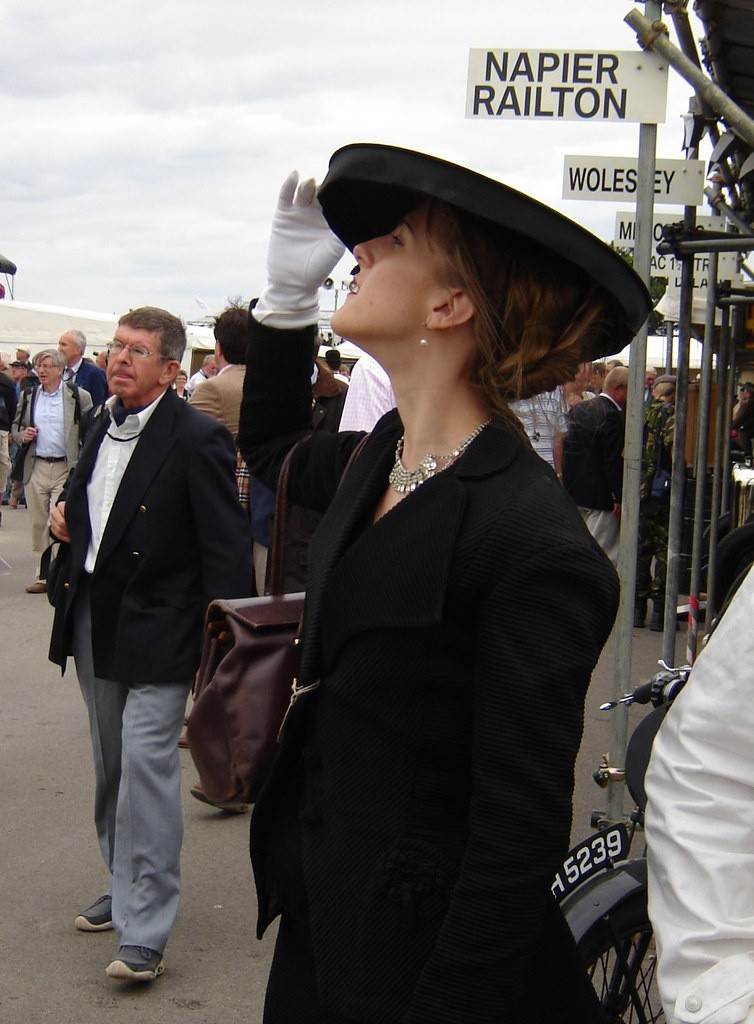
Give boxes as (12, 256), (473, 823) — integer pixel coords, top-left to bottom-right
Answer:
(650, 613), (681, 632)
(8, 495), (19, 508)
(26, 583), (47, 594)
(634, 612), (646, 628)
(191, 779), (249, 813)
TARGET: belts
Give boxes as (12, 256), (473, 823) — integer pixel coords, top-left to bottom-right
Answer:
(34, 454), (67, 463)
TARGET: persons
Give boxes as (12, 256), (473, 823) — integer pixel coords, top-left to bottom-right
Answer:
(0, 329), (113, 595)
(174, 309), (400, 698)
(230, 141), (651, 1024)
(642, 567), (753, 1024)
(508, 359), (754, 571)
(48, 306), (256, 983)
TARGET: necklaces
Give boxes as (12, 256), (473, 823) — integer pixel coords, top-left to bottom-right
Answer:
(390, 420), (492, 493)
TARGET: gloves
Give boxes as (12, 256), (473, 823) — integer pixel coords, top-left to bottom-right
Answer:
(250, 170), (347, 328)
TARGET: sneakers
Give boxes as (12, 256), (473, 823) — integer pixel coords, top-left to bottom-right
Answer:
(106, 945), (166, 980)
(76, 895), (113, 931)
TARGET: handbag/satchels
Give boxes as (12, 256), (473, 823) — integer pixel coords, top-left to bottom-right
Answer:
(9, 442), (31, 481)
(652, 465), (672, 500)
(184, 433), (370, 808)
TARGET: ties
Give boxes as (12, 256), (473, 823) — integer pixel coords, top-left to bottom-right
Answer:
(67, 369), (73, 384)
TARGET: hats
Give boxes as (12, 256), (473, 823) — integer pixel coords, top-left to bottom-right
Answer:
(15, 345), (31, 357)
(736, 381), (754, 391)
(9, 361), (28, 370)
(654, 375), (677, 387)
(324, 350), (342, 362)
(317, 144), (654, 364)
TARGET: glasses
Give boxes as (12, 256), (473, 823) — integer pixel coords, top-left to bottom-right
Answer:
(35, 362), (61, 370)
(106, 340), (169, 362)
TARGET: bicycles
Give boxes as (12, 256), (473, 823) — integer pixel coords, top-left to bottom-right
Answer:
(553, 657), (695, 1024)
